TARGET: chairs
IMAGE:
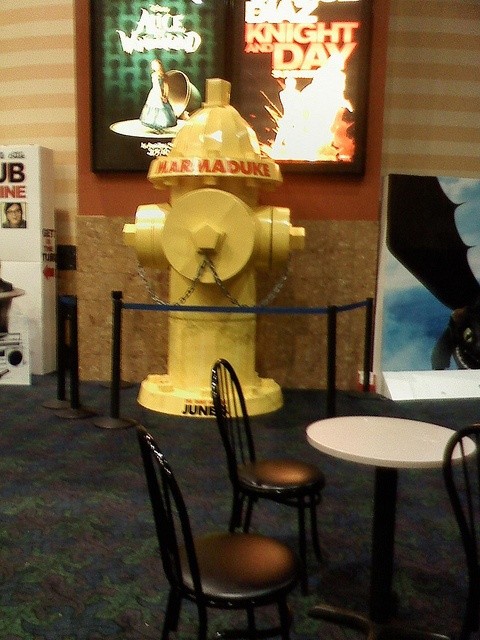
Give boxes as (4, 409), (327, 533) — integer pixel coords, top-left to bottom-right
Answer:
(442, 423), (479, 640)
(210, 358), (326, 595)
(135, 423), (299, 640)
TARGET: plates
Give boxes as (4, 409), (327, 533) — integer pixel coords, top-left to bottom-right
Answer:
(109, 117), (189, 147)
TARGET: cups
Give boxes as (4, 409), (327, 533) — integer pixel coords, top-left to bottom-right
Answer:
(164, 69), (203, 120)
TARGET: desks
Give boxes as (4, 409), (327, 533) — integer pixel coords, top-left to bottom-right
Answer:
(306, 415), (479, 639)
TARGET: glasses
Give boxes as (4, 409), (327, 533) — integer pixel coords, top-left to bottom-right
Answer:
(5, 209), (21, 214)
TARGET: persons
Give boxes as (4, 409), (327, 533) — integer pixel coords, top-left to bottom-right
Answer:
(2, 202), (27, 227)
(140, 60), (177, 134)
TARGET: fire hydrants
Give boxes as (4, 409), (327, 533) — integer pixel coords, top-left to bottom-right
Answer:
(121, 78), (305, 419)
